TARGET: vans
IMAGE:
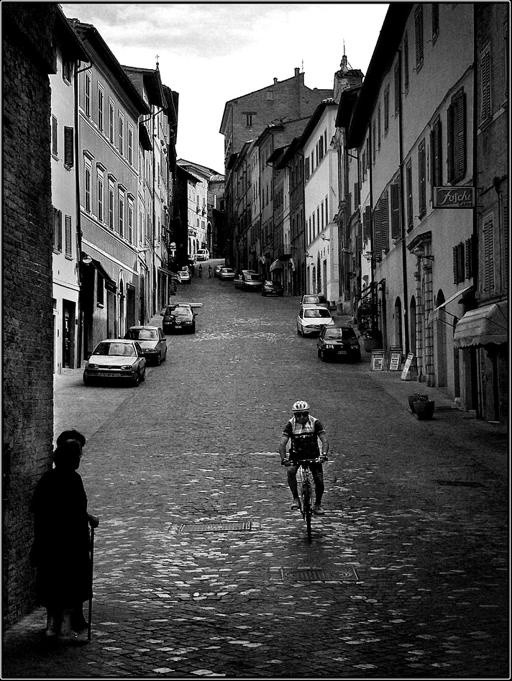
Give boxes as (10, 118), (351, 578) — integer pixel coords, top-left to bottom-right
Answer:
(235, 270), (262, 291)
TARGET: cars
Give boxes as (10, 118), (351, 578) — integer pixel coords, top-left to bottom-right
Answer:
(219, 267), (235, 280)
(176, 271), (193, 286)
(297, 293), (332, 315)
(158, 303), (199, 337)
(120, 324), (170, 368)
(213, 264), (224, 276)
(81, 338), (148, 389)
(261, 280), (284, 298)
(315, 325), (363, 362)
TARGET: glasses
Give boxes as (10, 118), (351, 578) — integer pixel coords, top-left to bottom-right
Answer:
(294, 410), (310, 417)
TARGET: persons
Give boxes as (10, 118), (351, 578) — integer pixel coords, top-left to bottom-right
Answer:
(26, 442), (91, 641)
(278, 400), (331, 518)
(56, 430), (101, 634)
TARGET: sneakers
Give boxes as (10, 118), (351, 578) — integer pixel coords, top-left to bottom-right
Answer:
(291, 497), (301, 510)
(314, 504), (325, 514)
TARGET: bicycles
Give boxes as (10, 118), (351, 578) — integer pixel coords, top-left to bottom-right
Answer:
(279, 457), (331, 541)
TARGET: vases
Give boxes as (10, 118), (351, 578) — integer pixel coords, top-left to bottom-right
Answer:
(413, 401), (434, 419)
(408, 395), (428, 413)
(364, 339), (379, 353)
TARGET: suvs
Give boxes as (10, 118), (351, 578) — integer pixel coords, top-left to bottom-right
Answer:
(196, 248), (211, 261)
(294, 307), (336, 338)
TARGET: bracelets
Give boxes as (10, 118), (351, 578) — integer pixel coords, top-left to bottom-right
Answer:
(322, 452), (328, 456)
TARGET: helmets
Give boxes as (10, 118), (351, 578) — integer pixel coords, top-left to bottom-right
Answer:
(292, 399), (310, 413)
